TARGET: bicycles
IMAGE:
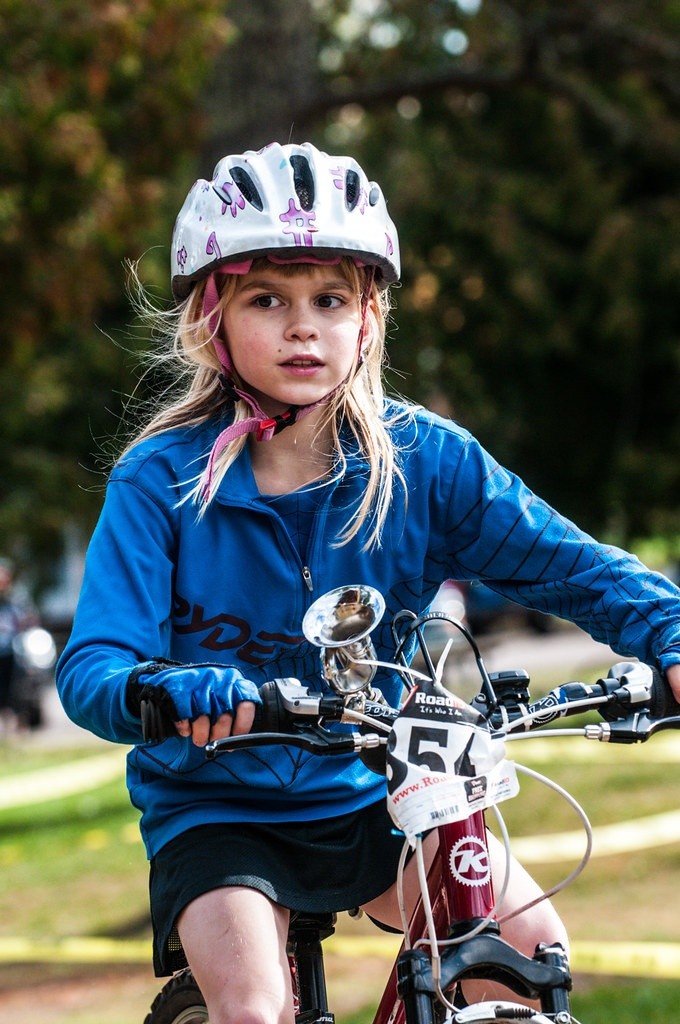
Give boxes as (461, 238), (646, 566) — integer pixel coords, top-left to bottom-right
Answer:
(136, 587), (680, 1024)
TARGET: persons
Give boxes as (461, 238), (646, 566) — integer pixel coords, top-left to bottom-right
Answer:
(1, 556), (46, 742)
(55, 142), (680, 1024)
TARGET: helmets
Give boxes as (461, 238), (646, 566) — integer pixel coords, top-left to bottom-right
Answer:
(170, 139), (400, 308)
